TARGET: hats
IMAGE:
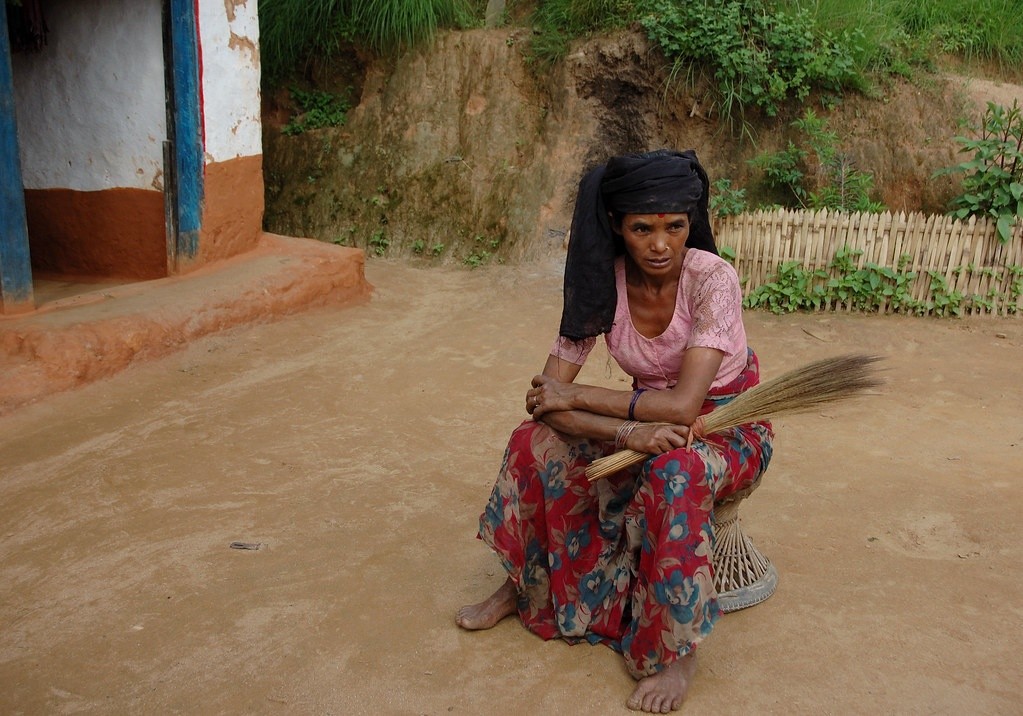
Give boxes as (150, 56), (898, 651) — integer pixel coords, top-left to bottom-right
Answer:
(559, 148), (721, 341)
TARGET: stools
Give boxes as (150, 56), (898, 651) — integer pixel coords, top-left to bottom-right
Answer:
(707, 470), (780, 616)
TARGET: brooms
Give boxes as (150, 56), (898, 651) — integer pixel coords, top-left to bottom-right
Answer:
(583, 350), (904, 486)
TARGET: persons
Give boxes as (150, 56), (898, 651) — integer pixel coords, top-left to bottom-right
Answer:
(455, 147), (773, 716)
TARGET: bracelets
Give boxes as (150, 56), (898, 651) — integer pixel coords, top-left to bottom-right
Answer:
(629, 388), (649, 419)
(614, 420), (640, 449)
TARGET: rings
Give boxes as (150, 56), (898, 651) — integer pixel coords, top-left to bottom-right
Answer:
(533, 394), (540, 405)
